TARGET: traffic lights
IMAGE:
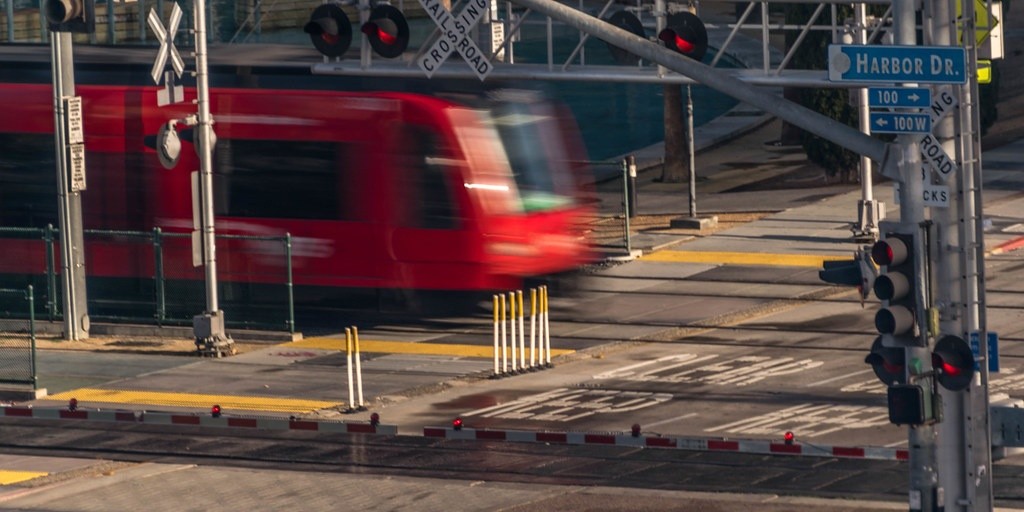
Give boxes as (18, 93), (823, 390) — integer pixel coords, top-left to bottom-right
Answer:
(39, 0), (97, 33)
(360, 2), (409, 60)
(931, 333), (975, 394)
(869, 220), (930, 350)
(818, 248), (875, 306)
(601, 13), (640, 64)
(657, 10), (706, 60)
(303, 4), (352, 56)
(888, 384), (935, 425)
(864, 337), (907, 388)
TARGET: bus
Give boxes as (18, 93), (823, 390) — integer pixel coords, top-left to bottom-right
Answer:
(0, 59), (607, 304)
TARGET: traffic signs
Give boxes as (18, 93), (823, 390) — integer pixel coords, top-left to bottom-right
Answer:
(869, 87), (931, 109)
(870, 112), (930, 136)
